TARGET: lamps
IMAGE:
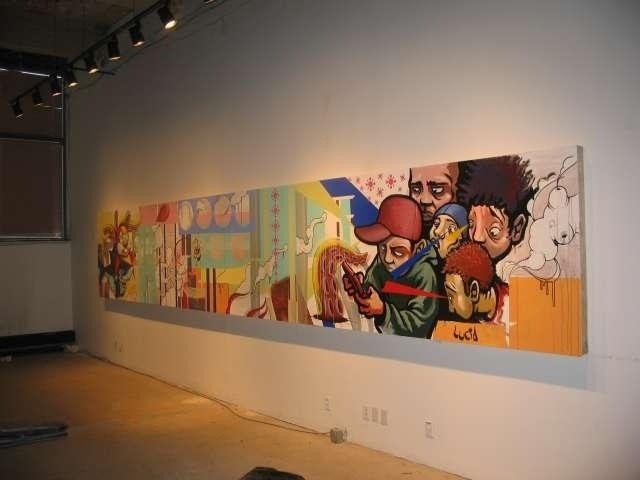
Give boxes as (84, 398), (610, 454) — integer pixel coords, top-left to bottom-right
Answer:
(7, 0), (179, 120)
